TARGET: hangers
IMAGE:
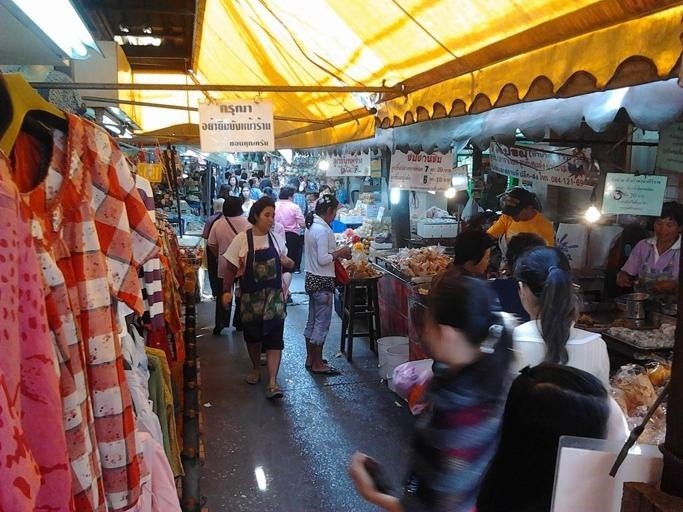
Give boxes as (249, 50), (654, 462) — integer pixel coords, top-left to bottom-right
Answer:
(0, 66), (92, 168)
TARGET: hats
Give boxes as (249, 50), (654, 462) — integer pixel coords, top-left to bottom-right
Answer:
(501, 188), (532, 216)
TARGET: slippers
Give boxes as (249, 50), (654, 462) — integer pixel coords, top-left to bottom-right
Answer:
(305, 359), (340, 375)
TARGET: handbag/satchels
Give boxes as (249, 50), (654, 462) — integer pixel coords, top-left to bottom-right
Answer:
(334, 258), (350, 287)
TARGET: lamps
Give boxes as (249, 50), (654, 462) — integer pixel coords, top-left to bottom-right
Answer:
(585, 190), (601, 224)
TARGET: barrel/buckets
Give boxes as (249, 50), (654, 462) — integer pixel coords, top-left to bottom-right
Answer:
(377, 336), (410, 381)
(385, 344), (409, 393)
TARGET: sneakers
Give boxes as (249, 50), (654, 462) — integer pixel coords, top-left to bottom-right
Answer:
(261, 353), (267, 366)
(266, 385), (284, 400)
(246, 370), (263, 385)
(212, 321), (229, 335)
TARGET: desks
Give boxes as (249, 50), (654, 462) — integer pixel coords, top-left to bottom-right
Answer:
(340, 275), (383, 362)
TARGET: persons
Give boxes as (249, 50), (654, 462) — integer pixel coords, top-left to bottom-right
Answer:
(348, 185), (683, 512)
(198, 162), (352, 397)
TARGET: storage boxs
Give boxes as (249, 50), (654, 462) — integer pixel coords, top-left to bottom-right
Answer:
(334, 287), (367, 320)
(368, 247), (390, 263)
(417, 221), (462, 239)
